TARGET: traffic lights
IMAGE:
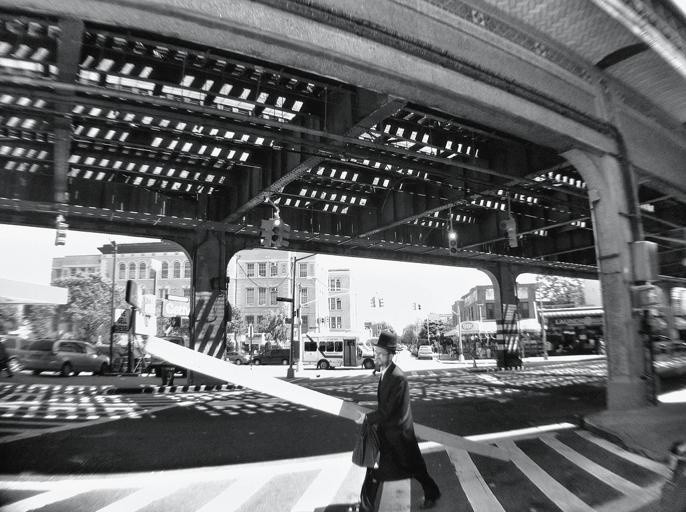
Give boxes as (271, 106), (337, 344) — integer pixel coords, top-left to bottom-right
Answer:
(448, 233), (460, 252)
(261, 219), (289, 253)
(501, 218), (520, 248)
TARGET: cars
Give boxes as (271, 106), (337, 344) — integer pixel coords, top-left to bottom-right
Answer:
(227, 349), (293, 366)
(367, 330), (381, 352)
(0, 329), (187, 380)
(652, 331), (673, 354)
(395, 344), (435, 360)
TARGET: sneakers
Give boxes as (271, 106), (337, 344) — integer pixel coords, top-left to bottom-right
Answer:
(419, 491), (441, 509)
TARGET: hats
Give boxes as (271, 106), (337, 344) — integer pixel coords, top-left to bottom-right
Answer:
(370, 329), (404, 352)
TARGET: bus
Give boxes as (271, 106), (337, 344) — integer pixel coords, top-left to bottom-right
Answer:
(301, 332), (380, 371)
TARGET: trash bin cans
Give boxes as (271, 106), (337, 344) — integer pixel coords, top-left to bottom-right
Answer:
(161, 365), (176, 386)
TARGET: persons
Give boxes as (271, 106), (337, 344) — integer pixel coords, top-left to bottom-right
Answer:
(349, 332), (441, 512)
